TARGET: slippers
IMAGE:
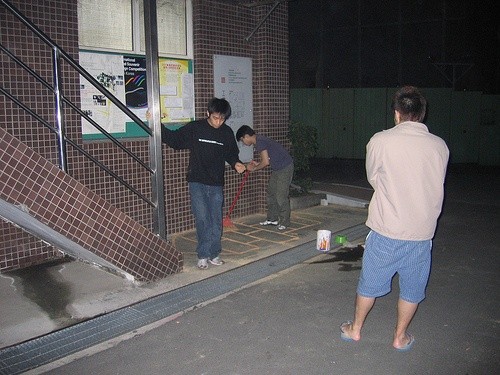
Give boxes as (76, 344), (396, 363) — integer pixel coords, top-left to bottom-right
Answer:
(340, 321), (352, 342)
(394, 333), (415, 351)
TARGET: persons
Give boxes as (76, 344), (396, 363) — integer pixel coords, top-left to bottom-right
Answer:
(146, 97), (247, 269)
(340, 86), (449, 352)
(236, 125), (294, 231)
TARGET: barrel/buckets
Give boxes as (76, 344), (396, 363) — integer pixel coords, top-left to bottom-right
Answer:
(317, 230), (331, 251)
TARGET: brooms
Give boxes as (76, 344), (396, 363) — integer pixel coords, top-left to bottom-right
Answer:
(223, 160), (255, 232)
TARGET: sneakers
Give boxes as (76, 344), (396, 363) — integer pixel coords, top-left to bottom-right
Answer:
(259, 220), (279, 226)
(198, 259), (208, 270)
(278, 225), (287, 231)
(208, 257), (225, 265)
